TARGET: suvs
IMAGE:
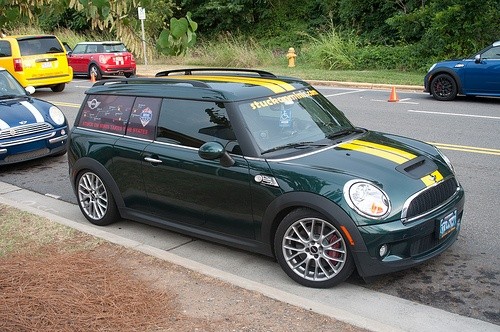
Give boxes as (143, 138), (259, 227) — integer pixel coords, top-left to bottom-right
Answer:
(0, 33), (74, 92)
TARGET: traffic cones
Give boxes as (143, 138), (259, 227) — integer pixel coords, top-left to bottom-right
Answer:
(387, 86), (400, 102)
(90, 72), (96, 83)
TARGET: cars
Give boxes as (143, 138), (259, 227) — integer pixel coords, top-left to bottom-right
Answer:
(62, 40), (136, 80)
(423, 41), (500, 101)
(65, 68), (465, 291)
(0, 67), (70, 165)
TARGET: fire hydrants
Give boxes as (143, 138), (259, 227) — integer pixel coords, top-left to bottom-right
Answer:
(285, 47), (297, 67)
